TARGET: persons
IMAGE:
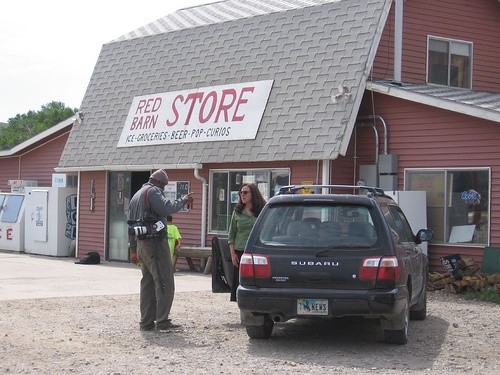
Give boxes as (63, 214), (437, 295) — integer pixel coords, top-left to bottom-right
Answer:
(127, 170), (193, 333)
(228, 183), (267, 326)
(456, 189), (480, 243)
(167, 215), (182, 273)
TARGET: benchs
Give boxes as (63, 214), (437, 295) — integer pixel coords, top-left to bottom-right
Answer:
(174, 247), (212, 275)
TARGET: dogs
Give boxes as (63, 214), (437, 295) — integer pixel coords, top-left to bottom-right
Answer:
(74, 252), (100, 264)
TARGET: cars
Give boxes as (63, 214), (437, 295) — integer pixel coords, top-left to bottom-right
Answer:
(450, 190), (482, 224)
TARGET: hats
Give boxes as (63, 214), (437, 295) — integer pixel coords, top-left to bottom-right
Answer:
(149, 169), (168, 185)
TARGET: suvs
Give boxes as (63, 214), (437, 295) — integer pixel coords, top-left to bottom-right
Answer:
(236, 184), (434, 345)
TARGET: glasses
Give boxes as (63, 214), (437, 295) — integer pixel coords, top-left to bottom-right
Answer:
(239, 191), (250, 195)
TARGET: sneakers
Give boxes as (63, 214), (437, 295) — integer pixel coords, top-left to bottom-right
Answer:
(140, 323), (184, 333)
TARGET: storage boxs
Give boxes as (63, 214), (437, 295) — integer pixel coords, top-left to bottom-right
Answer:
(440, 254), (473, 281)
(427, 271), (455, 291)
(462, 275), (487, 291)
(456, 257), (480, 276)
(483, 273), (500, 285)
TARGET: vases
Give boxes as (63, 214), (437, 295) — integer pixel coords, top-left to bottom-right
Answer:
(467, 212), (475, 225)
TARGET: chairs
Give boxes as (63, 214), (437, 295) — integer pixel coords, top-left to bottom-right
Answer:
(302, 217), (321, 233)
(348, 221), (374, 237)
(318, 220), (342, 239)
(287, 220), (313, 237)
